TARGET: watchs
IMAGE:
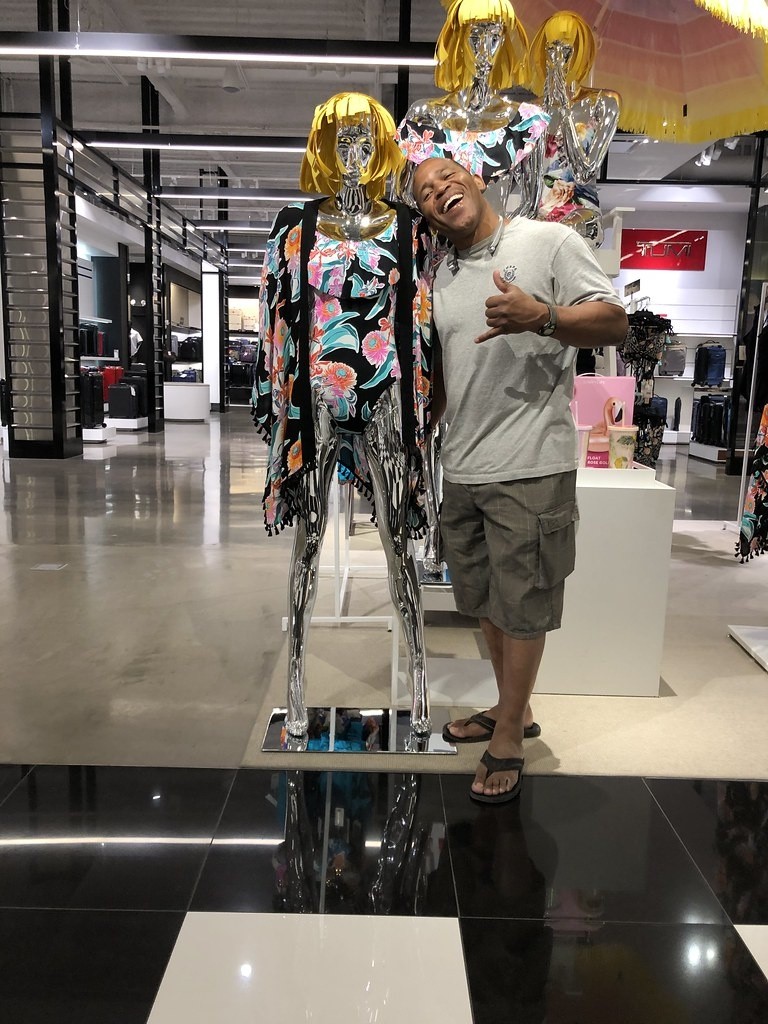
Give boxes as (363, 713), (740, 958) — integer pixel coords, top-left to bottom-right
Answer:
(538, 304), (557, 336)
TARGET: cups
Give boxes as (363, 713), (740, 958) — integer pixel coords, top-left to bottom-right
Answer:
(575, 424), (592, 467)
(607, 425), (639, 469)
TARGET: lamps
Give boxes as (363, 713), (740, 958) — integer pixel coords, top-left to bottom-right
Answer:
(156, 59), (166, 74)
(249, 180), (259, 188)
(695, 151), (706, 166)
(169, 178), (177, 186)
(306, 63), (318, 77)
(136, 58), (148, 71)
(724, 137), (740, 150)
(232, 179), (242, 188)
(241, 252), (247, 259)
(335, 64), (346, 78)
(713, 148), (721, 162)
(260, 212), (268, 222)
(252, 253), (258, 259)
(704, 145), (715, 166)
(222, 67), (241, 93)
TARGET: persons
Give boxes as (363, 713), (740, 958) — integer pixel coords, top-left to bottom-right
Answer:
(283, 768), (422, 915)
(531, 9), (622, 252)
(413, 157), (629, 803)
(394, 0), (552, 573)
(425, 797), (556, 1024)
(251, 91), (433, 735)
(130, 328), (144, 363)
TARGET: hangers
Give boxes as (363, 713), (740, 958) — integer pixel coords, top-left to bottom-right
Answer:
(635, 297), (657, 318)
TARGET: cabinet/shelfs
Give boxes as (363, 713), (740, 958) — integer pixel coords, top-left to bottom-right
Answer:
(79, 315), (119, 412)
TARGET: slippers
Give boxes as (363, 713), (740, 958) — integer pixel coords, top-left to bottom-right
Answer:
(443, 709), (540, 743)
(469, 749), (524, 804)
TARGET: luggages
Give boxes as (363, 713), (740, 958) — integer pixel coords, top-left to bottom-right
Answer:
(633, 394), (668, 427)
(633, 423), (665, 468)
(692, 393), (760, 450)
(77, 323), (259, 429)
(658, 340), (687, 376)
(691, 340), (726, 388)
